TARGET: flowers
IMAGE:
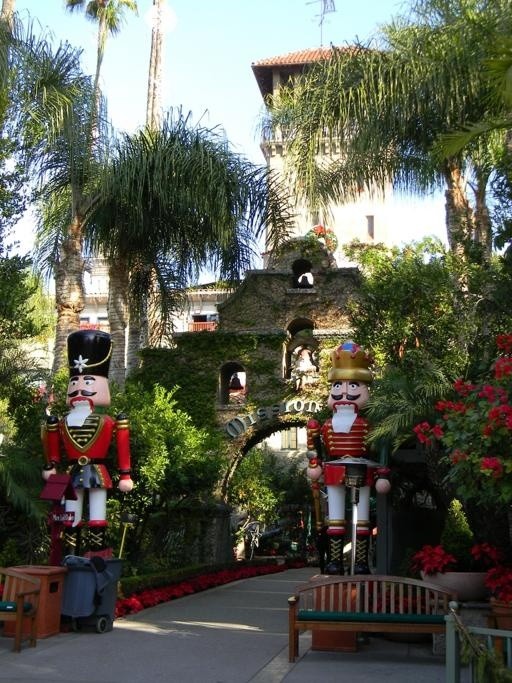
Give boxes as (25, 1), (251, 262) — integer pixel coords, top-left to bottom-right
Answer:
(415, 541), (498, 573)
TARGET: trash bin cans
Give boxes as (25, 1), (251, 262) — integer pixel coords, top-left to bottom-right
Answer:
(0, 555), (126, 639)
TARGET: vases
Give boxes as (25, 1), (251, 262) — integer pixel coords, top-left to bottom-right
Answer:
(419, 570), (487, 601)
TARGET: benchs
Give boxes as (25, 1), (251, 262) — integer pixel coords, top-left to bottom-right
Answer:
(286, 574), (465, 664)
(0, 573), (39, 654)
